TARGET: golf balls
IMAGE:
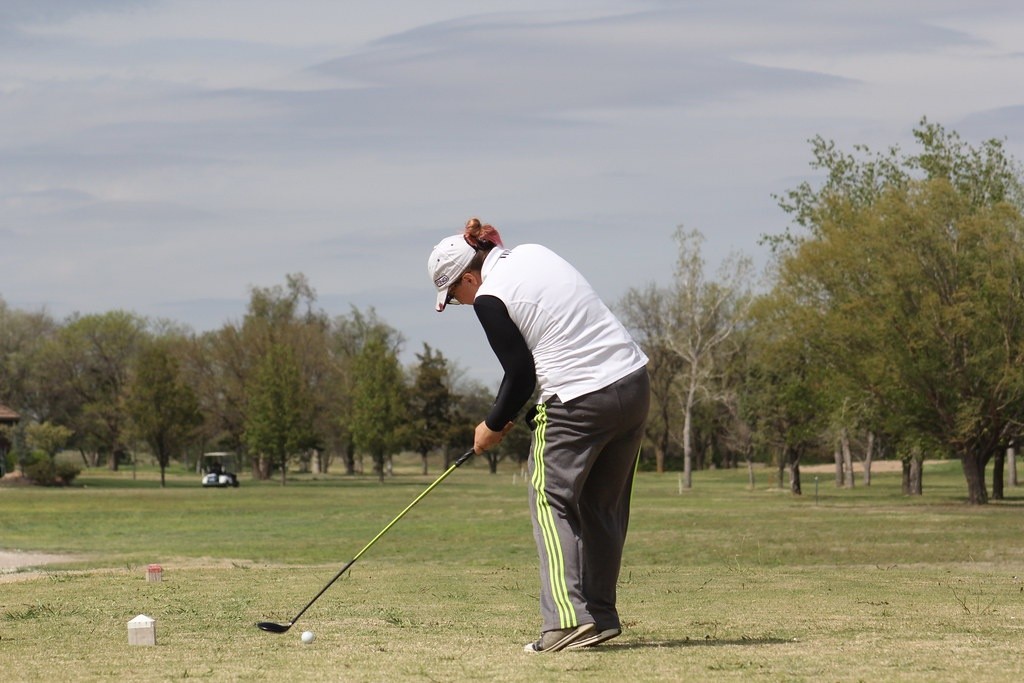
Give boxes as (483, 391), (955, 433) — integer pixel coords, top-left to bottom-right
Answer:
(301, 632), (314, 642)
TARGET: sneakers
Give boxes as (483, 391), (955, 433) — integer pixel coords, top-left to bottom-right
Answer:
(562, 628), (622, 649)
(523, 621), (594, 653)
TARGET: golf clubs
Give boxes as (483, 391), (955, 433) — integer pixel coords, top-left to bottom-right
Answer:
(256, 419), (516, 633)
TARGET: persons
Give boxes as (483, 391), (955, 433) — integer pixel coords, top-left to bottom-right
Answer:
(372, 441), (385, 484)
(428, 219), (650, 653)
(211, 462), (223, 475)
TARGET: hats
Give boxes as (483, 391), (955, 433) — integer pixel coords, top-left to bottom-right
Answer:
(428, 234), (477, 312)
